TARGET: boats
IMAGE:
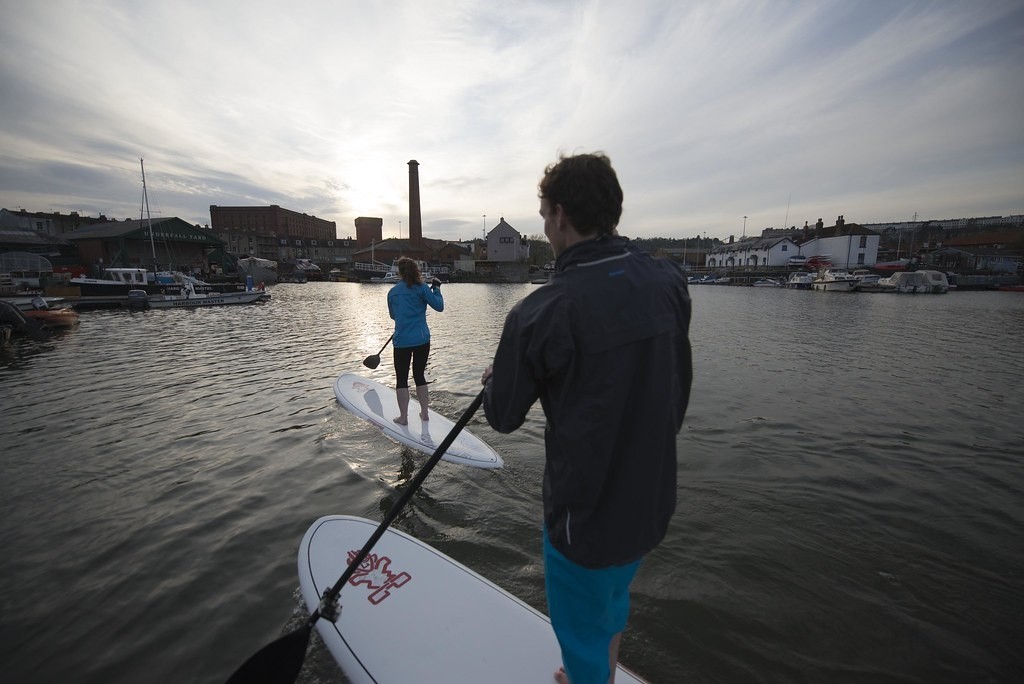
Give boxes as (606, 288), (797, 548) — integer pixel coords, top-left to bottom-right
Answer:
(753, 277), (781, 287)
(148, 291), (265, 308)
(384, 272), (434, 284)
(813, 269), (861, 291)
(878, 270), (949, 292)
(789, 273), (813, 289)
(853, 269), (881, 287)
(23, 307), (77, 331)
(687, 274), (730, 284)
(70, 159), (249, 297)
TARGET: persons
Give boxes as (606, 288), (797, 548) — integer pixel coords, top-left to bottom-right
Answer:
(482, 155), (693, 684)
(387, 257), (444, 425)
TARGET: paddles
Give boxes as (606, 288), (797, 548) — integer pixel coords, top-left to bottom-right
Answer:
(224, 386), (487, 684)
(362, 285), (434, 370)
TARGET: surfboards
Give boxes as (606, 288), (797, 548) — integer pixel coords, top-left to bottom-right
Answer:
(294, 513), (650, 684)
(332, 372), (505, 469)
